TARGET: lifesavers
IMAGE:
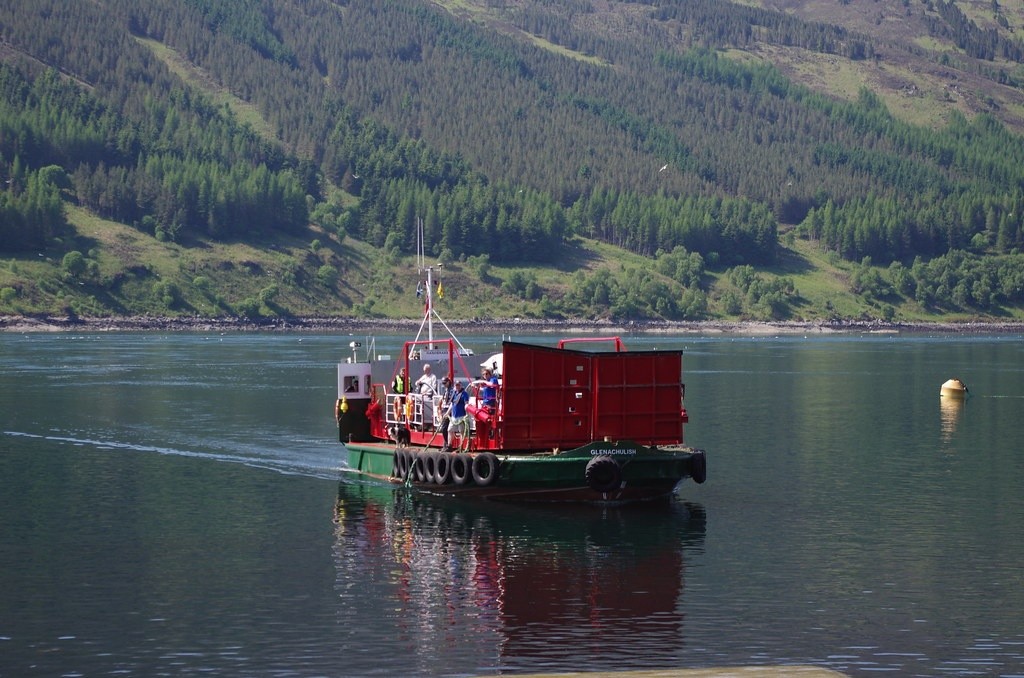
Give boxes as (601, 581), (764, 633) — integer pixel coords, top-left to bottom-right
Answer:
(451, 453), (473, 485)
(393, 448), (401, 475)
(434, 452), (453, 484)
(472, 452), (499, 487)
(585, 455), (623, 492)
(399, 450), (409, 480)
(693, 451), (706, 484)
(393, 397), (402, 420)
(415, 453), (428, 482)
(407, 451), (417, 480)
(425, 453), (440, 483)
(405, 396), (413, 421)
(437, 398), (443, 421)
(333, 399), (340, 422)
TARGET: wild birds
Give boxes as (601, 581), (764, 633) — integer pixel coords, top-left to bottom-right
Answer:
(352, 175), (359, 179)
(39, 253), (44, 257)
(518, 190), (523, 194)
(658, 164), (669, 173)
(5, 178), (14, 184)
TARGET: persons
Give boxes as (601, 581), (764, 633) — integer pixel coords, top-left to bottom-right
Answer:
(443, 381), (469, 453)
(414, 363), (437, 432)
(345, 376), (359, 392)
(392, 368), (413, 427)
(438, 376), (455, 452)
(478, 368), (498, 439)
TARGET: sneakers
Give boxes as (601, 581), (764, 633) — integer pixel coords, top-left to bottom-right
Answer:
(455, 448), (463, 453)
(443, 445), (453, 452)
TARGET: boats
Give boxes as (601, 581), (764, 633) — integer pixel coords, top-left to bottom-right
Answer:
(336, 216), (706, 511)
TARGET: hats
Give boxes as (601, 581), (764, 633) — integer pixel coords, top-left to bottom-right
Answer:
(455, 381), (461, 385)
(441, 377), (449, 383)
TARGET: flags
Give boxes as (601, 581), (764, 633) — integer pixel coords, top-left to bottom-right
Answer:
(416, 281), (423, 297)
